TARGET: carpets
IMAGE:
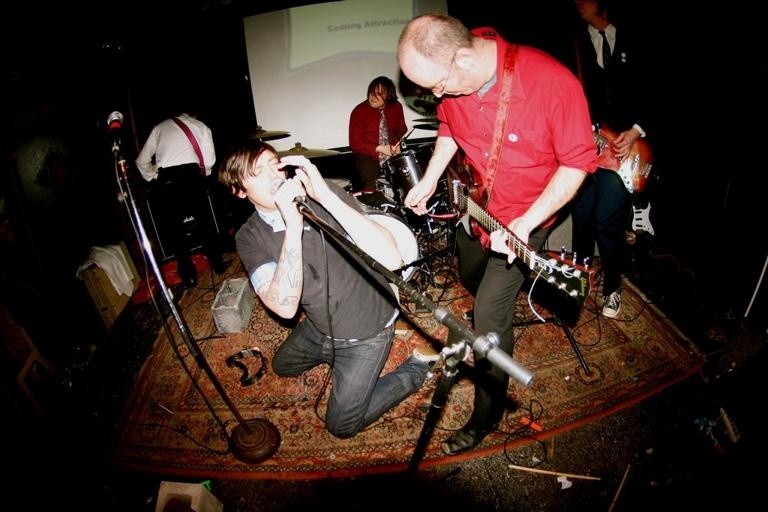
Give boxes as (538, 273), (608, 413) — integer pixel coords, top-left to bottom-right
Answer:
(110, 247), (711, 483)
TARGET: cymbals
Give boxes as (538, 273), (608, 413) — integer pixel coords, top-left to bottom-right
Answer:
(250, 131), (291, 138)
(413, 117), (441, 121)
(276, 148), (341, 158)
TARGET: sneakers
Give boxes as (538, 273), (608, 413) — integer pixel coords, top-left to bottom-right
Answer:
(412, 346), (441, 364)
(602, 287), (622, 319)
(461, 309), (474, 322)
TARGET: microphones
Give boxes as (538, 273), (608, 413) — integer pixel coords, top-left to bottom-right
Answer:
(274, 177), (304, 213)
(107, 111), (123, 158)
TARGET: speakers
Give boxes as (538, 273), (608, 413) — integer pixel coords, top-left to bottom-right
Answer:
(144, 179), (220, 259)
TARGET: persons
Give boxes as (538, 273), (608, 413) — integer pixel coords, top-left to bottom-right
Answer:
(349, 76), (408, 192)
(398, 14), (599, 455)
(218, 140), (440, 439)
(566, 0), (650, 317)
(134, 113), (232, 287)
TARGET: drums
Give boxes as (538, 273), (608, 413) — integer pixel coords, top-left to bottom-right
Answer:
(383, 143), (440, 206)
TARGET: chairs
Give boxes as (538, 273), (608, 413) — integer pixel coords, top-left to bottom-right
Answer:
(0, 306), (55, 418)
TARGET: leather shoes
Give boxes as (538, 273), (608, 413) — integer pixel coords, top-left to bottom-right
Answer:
(183, 260), (231, 289)
(440, 416), (498, 456)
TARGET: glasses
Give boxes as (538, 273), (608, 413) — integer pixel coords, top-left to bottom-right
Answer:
(431, 52), (456, 95)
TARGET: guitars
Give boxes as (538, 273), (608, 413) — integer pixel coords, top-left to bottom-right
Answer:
(593, 121), (653, 193)
(447, 164), (594, 301)
(626, 201), (657, 244)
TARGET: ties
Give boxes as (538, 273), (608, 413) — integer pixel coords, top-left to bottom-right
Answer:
(378, 110), (391, 169)
(598, 30), (612, 68)
